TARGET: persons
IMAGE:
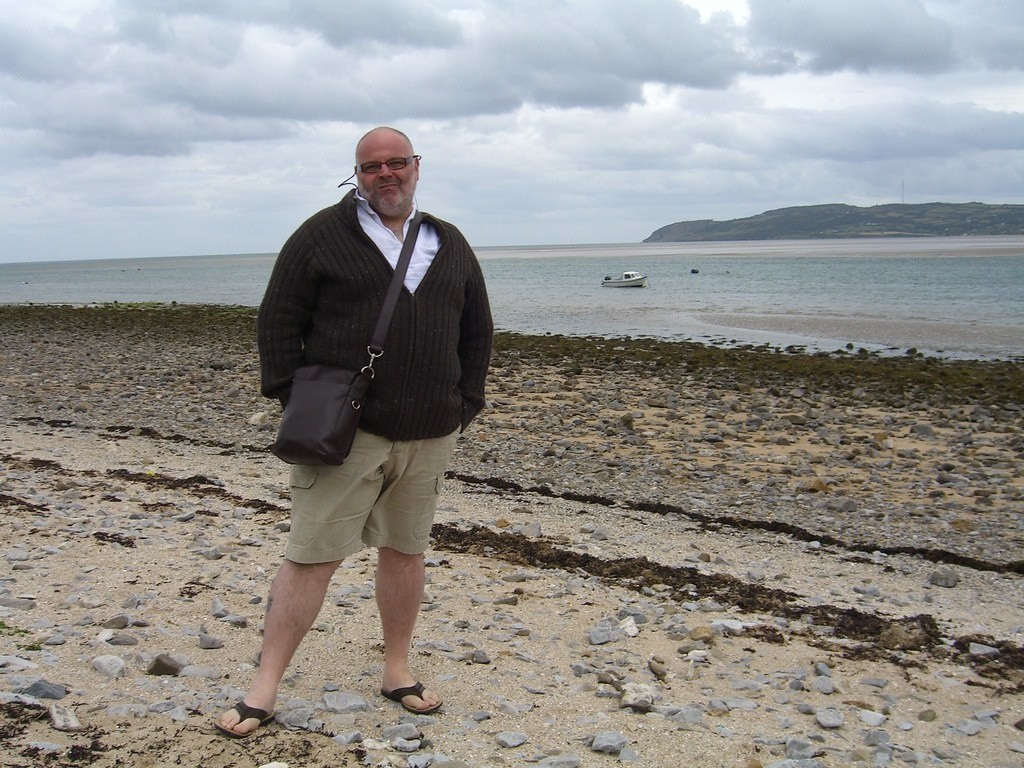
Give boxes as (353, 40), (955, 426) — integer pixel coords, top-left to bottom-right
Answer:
(218, 126), (493, 737)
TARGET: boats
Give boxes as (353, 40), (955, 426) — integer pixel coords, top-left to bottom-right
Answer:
(602, 271), (647, 287)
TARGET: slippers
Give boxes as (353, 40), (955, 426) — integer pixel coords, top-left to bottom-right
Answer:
(380, 680), (443, 714)
(214, 701), (275, 737)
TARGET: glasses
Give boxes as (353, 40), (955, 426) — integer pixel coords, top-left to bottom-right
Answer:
(357, 155), (422, 173)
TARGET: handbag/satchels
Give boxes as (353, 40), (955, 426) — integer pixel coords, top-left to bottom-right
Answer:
(271, 365), (378, 465)
